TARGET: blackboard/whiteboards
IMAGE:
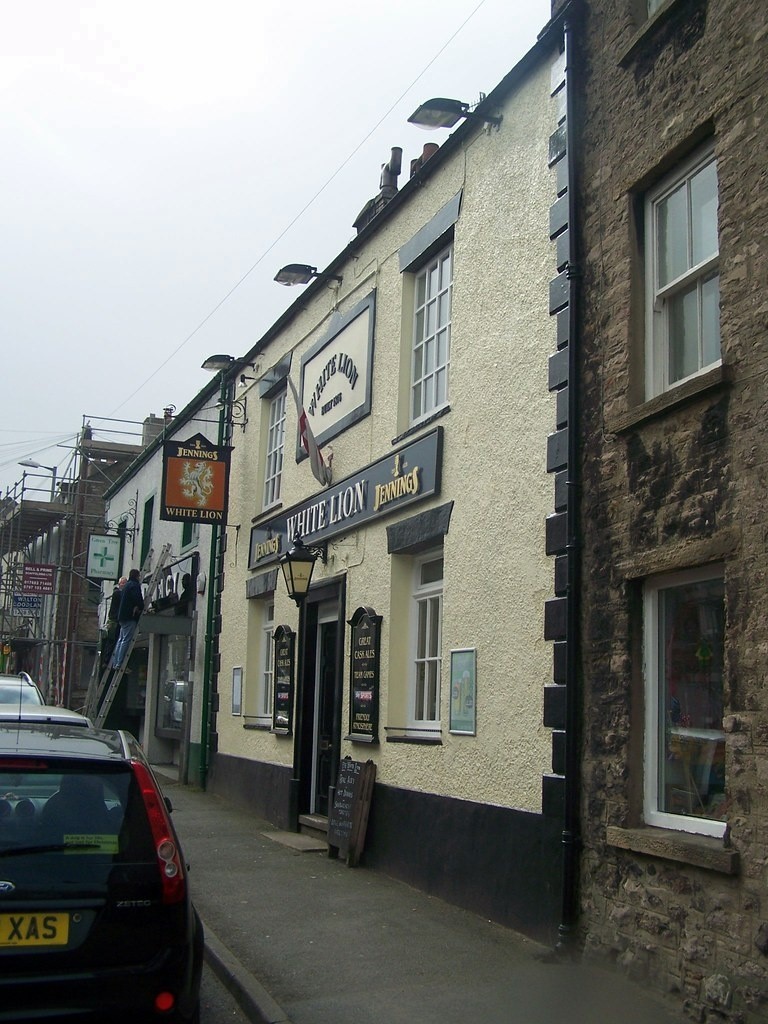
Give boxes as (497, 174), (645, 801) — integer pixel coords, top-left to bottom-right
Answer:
(329, 756), (376, 853)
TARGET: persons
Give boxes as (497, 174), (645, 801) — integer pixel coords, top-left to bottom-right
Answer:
(180, 572), (192, 600)
(102, 575), (128, 669)
(113, 569), (143, 674)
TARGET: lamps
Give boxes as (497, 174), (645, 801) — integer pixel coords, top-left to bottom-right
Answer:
(272, 263), (343, 288)
(201, 353), (258, 374)
(405, 98), (506, 133)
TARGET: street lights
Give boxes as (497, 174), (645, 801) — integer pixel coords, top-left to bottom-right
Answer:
(16, 457), (57, 683)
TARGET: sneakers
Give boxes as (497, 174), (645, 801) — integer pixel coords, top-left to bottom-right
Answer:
(114, 664), (132, 674)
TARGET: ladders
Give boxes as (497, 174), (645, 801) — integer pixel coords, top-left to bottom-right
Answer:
(95, 543), (174, 729)
(87, 548), (155, 721)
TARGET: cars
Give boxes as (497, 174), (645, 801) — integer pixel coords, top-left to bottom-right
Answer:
(1, 670), (44, 705)
(162, 680), (187, 729)
(0, 721), (204, 1023)
